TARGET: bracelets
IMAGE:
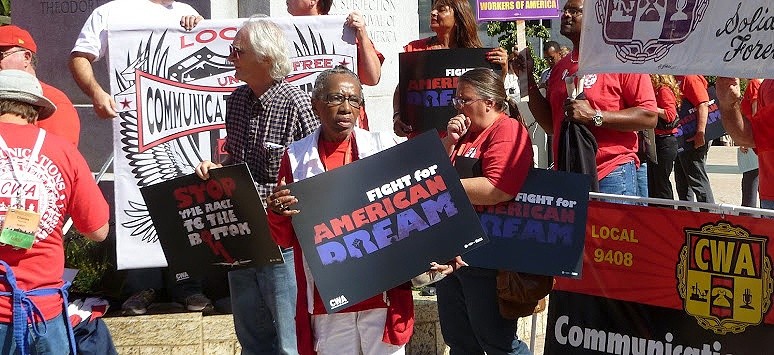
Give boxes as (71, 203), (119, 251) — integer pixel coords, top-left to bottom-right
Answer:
(393, 111), (399, 119)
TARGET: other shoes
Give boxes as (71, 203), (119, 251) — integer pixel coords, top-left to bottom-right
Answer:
(420, 287), (436, 295)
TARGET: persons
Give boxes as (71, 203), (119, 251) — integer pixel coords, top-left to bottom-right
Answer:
(286, 0), (385, 131)
(647, 74), (684, 209)
(435, 67), (534, 355)
(0, 25), (81, 147)
(674, 75), (717, 213)
(0, 69), (109, 355)
(636, 128), (658, 206)
(715, 77), (774, 218)
(70, 0), (214, 316)
(265, 63), (469, 355)
(195, 21), (321, 355)
(538, 42), (563, 169)
(393, 0), (508, 138)
(509, 0), (658, 205)
(738, 78), (763, 216)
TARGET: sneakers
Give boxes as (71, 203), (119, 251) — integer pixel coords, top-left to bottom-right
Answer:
(175, 290), (214, 311)
(121, 288), (162, 315)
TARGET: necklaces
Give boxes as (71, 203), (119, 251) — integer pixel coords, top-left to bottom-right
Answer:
(570, 51), (578, 63)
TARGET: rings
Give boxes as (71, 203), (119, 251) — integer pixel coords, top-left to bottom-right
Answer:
(356, 18), (358, 22)
(281, 204), (285, 209)
(275, 198), (280, 204)
(452, 120), (454, 124)
(499, 56), (501, 60)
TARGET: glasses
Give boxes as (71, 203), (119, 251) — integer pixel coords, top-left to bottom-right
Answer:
(229, 45), (241, 53)
(0, 49), (24, 60)
(561, 9), (582, 14)
(452, 97), (488, 107)
(317, 93), (361, 108)
(544, 52), (558, 61)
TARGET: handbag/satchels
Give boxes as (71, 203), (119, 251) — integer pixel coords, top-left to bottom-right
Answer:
(496, 270), (553, 320)
(654, 85), (680, 130)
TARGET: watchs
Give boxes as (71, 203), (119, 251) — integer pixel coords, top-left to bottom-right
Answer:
(593, 110), (603, 126)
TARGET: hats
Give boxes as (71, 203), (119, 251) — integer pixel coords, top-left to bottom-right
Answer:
(0, 25), (36, 53)
(0, 69), (57, 121)
